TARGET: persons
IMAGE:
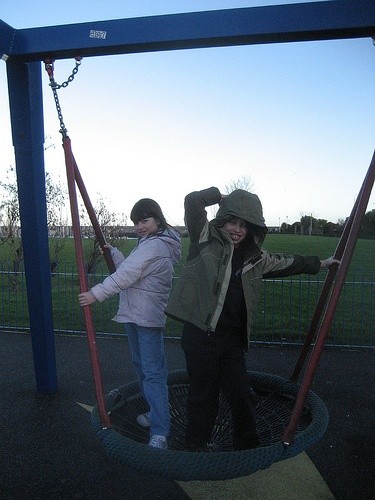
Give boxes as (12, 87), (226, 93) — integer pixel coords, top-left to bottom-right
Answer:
(164, 187), (341, 453)
(78, 198), (183, 451)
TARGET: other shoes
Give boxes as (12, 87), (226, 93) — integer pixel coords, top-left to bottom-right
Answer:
(175, 433), (208, 452)
(137, 412), (152, 427)
(148, 434), (169, 450)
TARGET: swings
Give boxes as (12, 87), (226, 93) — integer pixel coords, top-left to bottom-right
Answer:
(44, 58), (374, 481)
